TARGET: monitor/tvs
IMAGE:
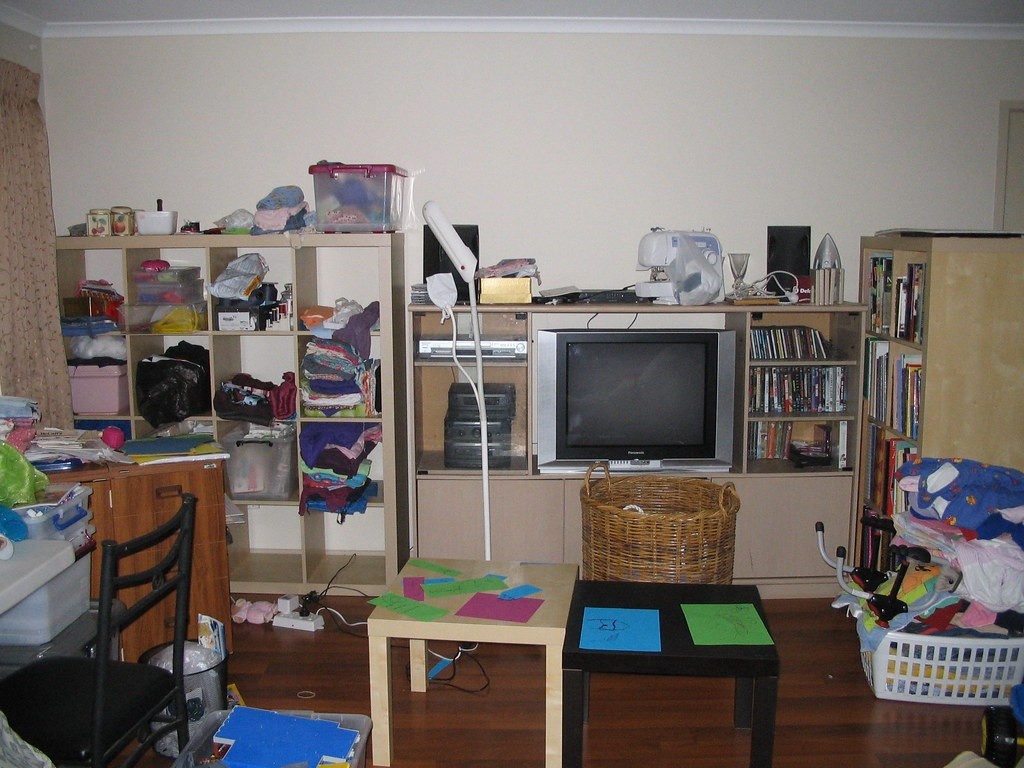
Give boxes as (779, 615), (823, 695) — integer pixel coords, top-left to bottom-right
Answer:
(536, 328), (737, 475)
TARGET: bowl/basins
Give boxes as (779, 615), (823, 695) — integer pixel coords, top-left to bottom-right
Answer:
(135, 211), (178, 235)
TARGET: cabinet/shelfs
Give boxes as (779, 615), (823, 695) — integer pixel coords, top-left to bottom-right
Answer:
(58, 232), (400, 593)
(406, 305), (873, 560)
(46, 451), (236, 659)
(858, 235), (1024, 571)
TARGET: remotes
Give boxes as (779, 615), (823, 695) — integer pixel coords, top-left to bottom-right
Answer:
(592, 458), (662, 471)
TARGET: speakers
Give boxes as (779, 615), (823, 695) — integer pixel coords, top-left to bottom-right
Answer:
(422, 225), (479, 305)
(766, 226), (811, 302)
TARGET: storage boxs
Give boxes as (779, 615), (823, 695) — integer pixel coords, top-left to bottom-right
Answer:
(170, 704), (372, 768)
(0, 597), (126, 681)
(132, 267), (202, 286)
(138, 282), (205, 304)
(70, 361), (128, 413)
(310, 163), (405, 233)
(479, 279), (530, 301)
(0, 483), (93, 645)
(226, 428), (291, 499)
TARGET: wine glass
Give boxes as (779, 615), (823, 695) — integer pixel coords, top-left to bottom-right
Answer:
(729, 253), (749, 288)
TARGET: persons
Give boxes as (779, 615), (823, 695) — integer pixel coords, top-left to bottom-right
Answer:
(200, 635), (214, 649)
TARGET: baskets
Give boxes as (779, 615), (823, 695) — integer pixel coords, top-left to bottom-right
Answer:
(581, 461), (740, 585)
(861, 615), (1024, 706)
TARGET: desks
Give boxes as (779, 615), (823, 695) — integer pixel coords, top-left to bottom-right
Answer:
(563, 578), (781, 768)
(363, 556), (579, 768)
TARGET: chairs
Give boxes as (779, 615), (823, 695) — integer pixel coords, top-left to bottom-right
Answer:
(0, 494), (200, 768)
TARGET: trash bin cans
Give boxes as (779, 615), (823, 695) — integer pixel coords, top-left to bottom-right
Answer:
(137, 639), (228, 759)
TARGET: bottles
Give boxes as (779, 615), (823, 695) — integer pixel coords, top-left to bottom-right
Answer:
(259, 282), (279, 301)
(110, 207), (134, 236)
(86, 209), (111, 237)
(282, 284), (293, 316)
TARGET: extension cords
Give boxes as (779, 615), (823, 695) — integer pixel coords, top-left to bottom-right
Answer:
(272, 611), (324, 631)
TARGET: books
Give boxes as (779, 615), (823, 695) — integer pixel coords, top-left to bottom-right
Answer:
(11, 482), (80, 510)
(196, 613), (226, 661)
(224, 492), (245, 524)
(747, 326), (848, 469)
(858, 248), (925, 572)
(30, 427), (230, 466)
